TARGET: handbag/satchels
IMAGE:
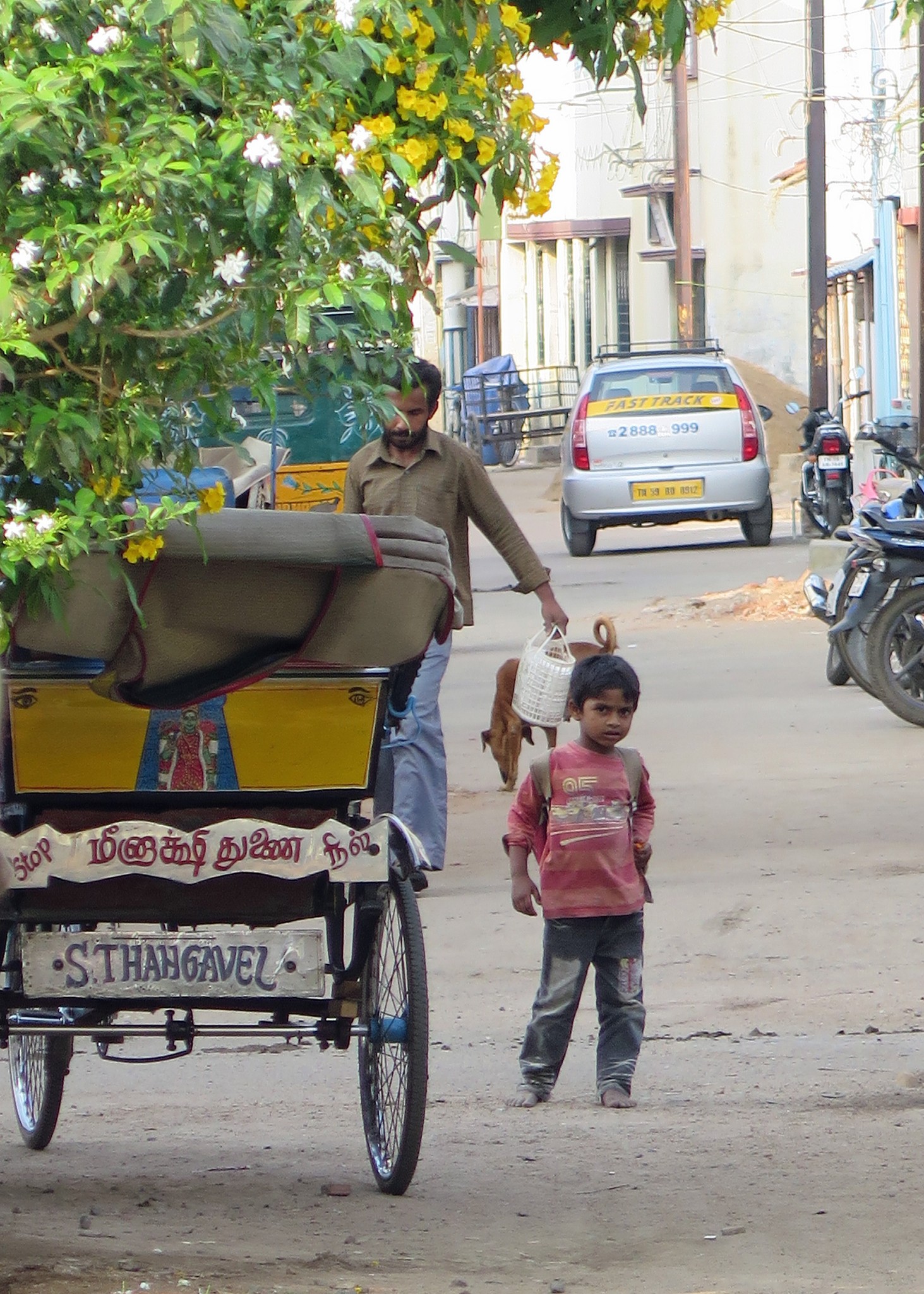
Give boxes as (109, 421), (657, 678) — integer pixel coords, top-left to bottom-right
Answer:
(511, 624), (577, 729)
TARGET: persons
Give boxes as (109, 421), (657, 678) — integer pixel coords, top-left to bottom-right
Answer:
(346, 355), (569, 894)
(500, 655), (656, 1108)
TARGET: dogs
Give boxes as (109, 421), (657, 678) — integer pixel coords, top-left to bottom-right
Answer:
(479, 615), (620, 792)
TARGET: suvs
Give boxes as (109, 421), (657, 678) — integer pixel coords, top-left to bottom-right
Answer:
(560, 338), (773, 557)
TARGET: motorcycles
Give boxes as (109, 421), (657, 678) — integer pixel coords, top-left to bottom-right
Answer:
(784, 366), (871, 535)
(758, 415), (924, 579)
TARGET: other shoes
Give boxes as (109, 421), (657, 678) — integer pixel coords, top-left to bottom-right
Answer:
(408, 871), (428, 893)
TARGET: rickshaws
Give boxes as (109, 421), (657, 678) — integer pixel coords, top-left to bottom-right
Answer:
(1, 505), (457, 1195)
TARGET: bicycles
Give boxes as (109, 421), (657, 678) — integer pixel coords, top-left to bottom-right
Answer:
(442, 383), (524, 467)
